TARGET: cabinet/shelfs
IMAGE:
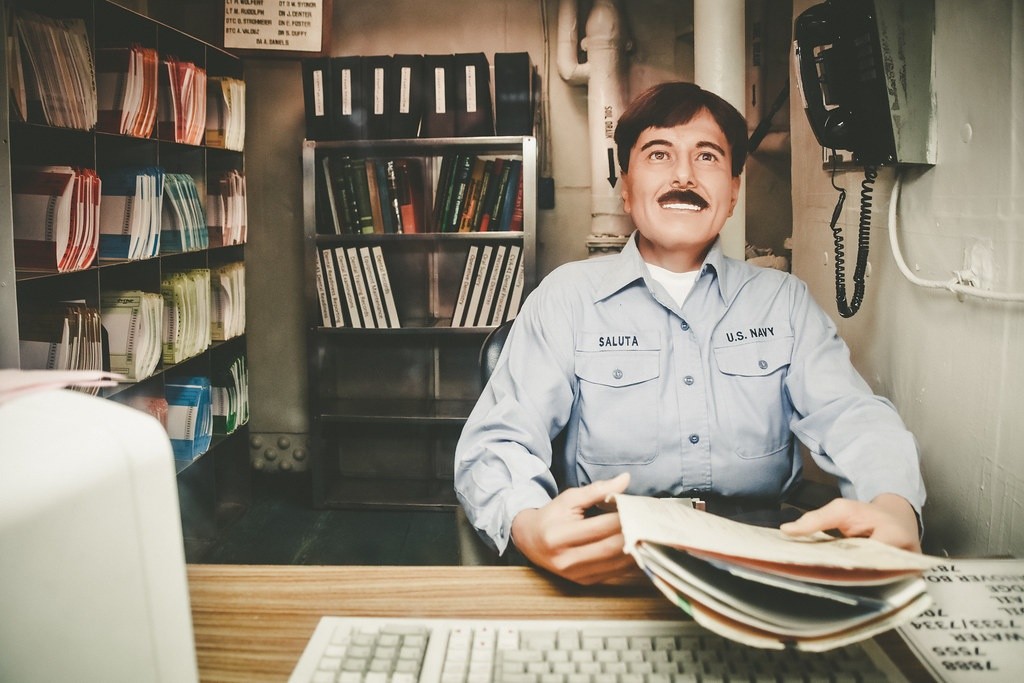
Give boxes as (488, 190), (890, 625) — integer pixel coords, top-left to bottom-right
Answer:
(0, 0), (248, 545)
(299, 50), (542, 517)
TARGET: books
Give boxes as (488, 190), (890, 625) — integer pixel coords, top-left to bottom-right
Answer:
(316, 148), (525, 233)
(7, 5), (250, 459)
(451, 241), (524, 328)
(596, 494), (951, 653)
(313, 244), (404, 330)
(300, 52), (537, 143)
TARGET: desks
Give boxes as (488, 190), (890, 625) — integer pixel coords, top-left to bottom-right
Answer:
(184, 552), (1024, 683)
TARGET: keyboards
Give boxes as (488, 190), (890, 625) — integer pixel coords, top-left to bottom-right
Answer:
(287, 614), (909, 683)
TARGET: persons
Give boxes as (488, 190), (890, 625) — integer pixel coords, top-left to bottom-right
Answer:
(454, 80), (926, 588)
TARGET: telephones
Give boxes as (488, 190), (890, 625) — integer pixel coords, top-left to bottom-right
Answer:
(793, 0), (898, 167)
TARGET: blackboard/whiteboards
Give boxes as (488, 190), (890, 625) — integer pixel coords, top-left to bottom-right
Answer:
(219, 0), (333, 60)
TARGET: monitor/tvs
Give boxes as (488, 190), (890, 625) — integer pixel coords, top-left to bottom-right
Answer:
(0, 389), (201, 683)
